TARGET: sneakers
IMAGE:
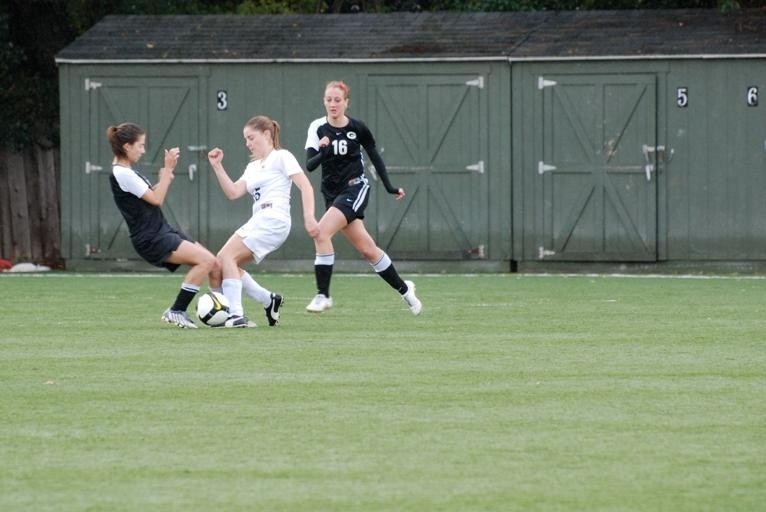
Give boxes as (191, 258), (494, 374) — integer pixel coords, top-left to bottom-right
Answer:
(162, 308), (198, 329)
(264, 293), (284, 326)
(306, 294), (332, 311)
(211, 315), (248, 328)
(401, 280), (422, 315)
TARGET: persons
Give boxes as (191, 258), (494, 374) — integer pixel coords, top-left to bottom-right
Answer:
(305, 82), (423, 317)
(106, 123), (257, 328)
(208, 116), (321, 328)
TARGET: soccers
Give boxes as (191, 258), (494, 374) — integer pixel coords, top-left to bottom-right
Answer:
(196, 291), (230, 325)
